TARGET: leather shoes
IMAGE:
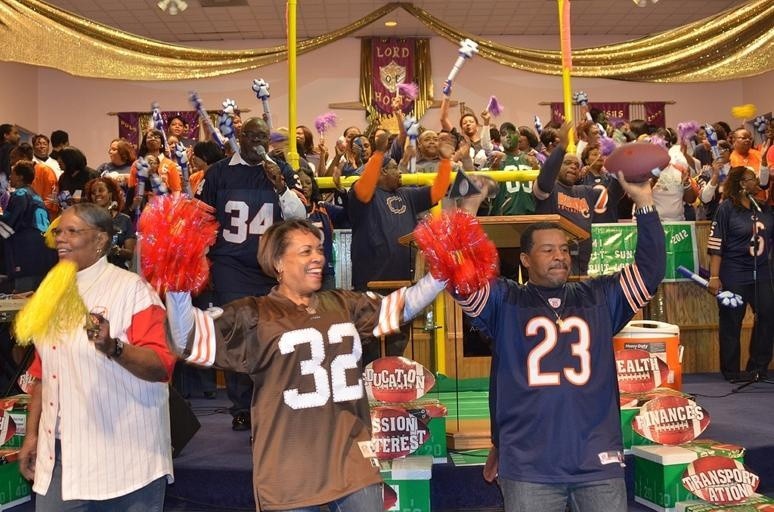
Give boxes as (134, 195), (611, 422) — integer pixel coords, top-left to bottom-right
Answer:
(231, 413), (252, 431)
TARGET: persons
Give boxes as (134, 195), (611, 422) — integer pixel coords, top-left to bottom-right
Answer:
(1, 81), (773, 456)
(165, 217), (451, 512)
(444, 169), (668, 512)
(17, 203), (176, 512)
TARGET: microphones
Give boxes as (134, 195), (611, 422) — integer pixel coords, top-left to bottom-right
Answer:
(255, 145), (278, 166)
(745, 191), (764, 215)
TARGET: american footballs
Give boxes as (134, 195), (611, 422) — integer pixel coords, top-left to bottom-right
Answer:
(0, 409), (16, 449)
(604, 144), (670, 184)
(633, 394), (710, 446)
(363, 357), (435, 404)
(613, 349), (670, 397)
(681, 456), (762, 505)
(355, 409), (427, 462)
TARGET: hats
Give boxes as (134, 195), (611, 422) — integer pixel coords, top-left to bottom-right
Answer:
(500, 122), (516, 132)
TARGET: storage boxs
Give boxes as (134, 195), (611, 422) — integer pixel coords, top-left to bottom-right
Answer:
(369, 399), (450, 463)
(0, 447), (33, 509)
(611, 320), (681, 407)
(676, 492), (774, 512)
(375, 455), (432, 512)
(1, 394), (37, 448)
(620, 394), (695, 455)
(631, 439), (746, 512)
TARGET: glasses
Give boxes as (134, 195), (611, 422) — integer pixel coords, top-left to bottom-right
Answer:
(50, 227), (91, 237)
(244, 131), (267, 140)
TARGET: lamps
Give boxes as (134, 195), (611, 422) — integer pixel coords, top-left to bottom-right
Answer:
(158, 1), (188, 16)
